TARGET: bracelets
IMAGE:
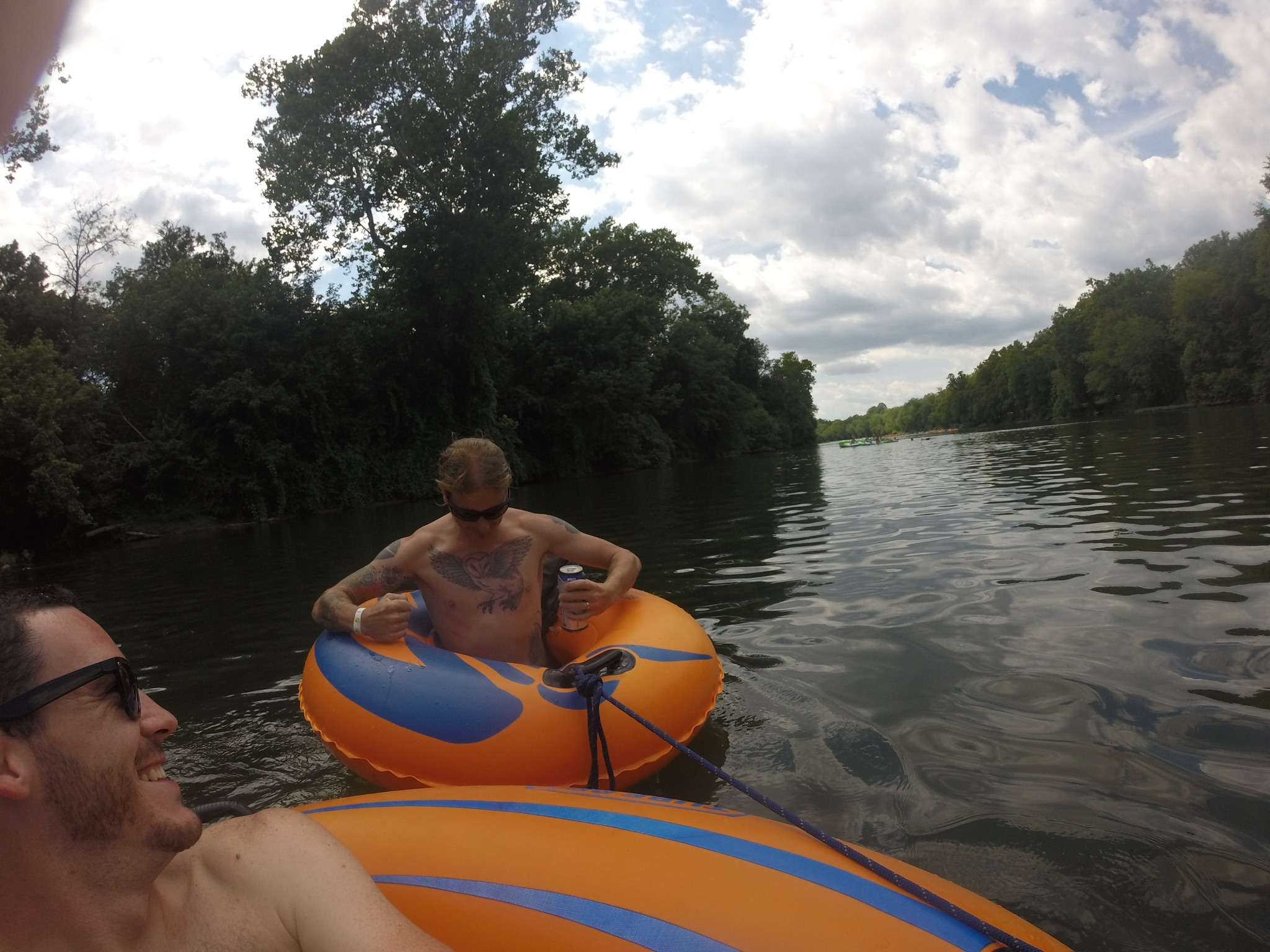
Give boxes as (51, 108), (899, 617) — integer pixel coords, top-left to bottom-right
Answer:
(351, 606), (366, 639)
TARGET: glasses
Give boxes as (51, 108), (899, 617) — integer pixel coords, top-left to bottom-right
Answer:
(0, 656), (145, 723)
(445, 486), (511, 522)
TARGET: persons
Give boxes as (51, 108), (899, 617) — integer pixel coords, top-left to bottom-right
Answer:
(0, 562), (467, 952)
(310, 437), (643, 670)
